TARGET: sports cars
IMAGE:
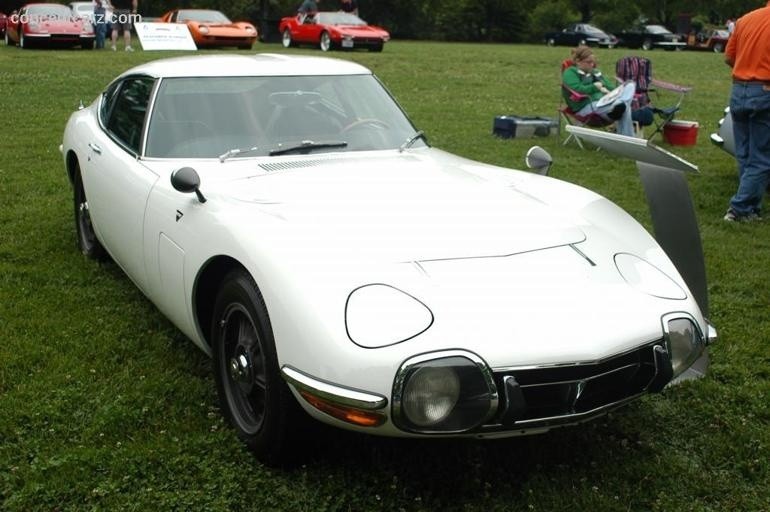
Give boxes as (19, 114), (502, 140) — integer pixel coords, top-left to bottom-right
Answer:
(4, 2), (96, 49)
(154, 9), (258, 50)
(279, 11), (391, 54)
(56, 57), (715, 463)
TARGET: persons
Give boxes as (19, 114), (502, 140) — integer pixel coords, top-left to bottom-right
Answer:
(91, 1), (107, 48)
(109, 0), (139, 52)
(561, 44), (636, 136)
(296, 0), (321, 21)
(722, 1), (770, 222)
(725, 18), (735, 38)
(338, 0), (359, 18)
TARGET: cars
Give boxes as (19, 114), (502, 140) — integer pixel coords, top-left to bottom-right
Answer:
(546, 22), (733, 54)
(67, 1), (94, 28)
(0, 12), (10, 37)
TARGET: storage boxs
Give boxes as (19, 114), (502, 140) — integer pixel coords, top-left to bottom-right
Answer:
(662, 119), (699, 146)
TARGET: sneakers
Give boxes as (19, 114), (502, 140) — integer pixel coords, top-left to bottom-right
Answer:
(723, 205), (762, 223)
(606, 101), (625, 121)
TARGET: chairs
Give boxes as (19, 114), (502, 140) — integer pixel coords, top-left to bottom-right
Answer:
(613, 56), (693, 146)
(558, 60), (641, 154)
